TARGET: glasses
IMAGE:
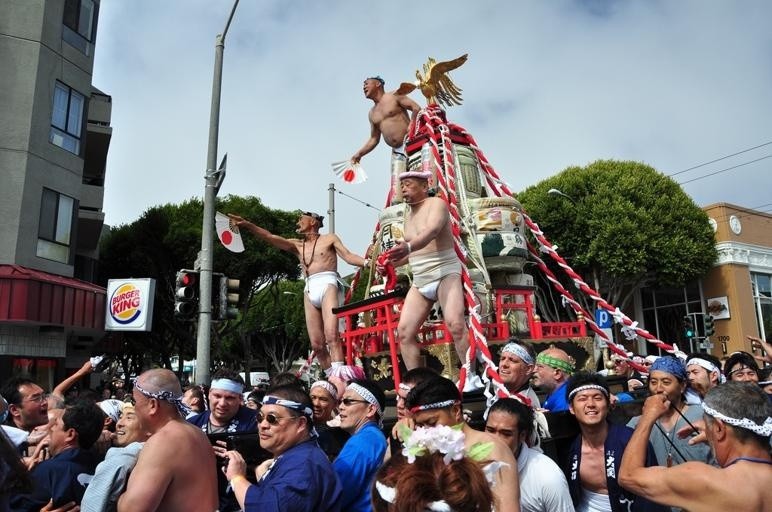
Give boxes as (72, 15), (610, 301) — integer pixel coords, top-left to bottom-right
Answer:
(337, 398), (371, 407)
(15, 392), (50, 404)
(129, 396), (146, 407)
(256, 412), (302, 425)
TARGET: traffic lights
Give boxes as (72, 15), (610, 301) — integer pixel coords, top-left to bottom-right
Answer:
(219, 276), (241, 322)
(172, 269), (197, 319)
(682, 314), (695, 339)
(703, 315), (716, 338)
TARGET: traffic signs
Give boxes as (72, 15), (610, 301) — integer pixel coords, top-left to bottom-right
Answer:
(700, 343), (714, 349)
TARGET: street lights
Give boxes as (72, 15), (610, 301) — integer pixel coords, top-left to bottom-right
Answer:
(544, 187), (612, 370)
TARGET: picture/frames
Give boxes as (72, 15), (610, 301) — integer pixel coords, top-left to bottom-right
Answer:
(706, 295), (731, 321)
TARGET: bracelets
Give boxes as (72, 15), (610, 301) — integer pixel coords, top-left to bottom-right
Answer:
(407, 242), (411, 252)
(363, 259), (369, 267)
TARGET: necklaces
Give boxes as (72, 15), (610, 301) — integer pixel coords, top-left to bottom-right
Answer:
(406, 197), (429, 205)
(303, 234), (320, 277)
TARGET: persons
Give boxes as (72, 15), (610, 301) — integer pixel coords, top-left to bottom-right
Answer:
(227, 210), (385, 379)
(386, 169), (482, 391)
(351, 76), (421, 206)
(2, 336), (772, 511)
